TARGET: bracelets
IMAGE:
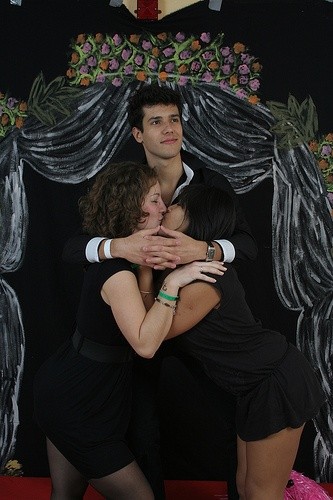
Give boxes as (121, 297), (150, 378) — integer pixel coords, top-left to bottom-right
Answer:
(155, 298), (177, 312)
(155, 289), (181, 300)
(103, 238), (114, 261)
(140, 286), (152, 296)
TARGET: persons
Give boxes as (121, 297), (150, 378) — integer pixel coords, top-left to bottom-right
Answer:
(45, 162), (222, 500)
(139, 183), (326, 500)
(62, 85), (258, 500)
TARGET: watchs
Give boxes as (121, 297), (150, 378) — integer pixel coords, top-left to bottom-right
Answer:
(206, 242), (217, 264)
